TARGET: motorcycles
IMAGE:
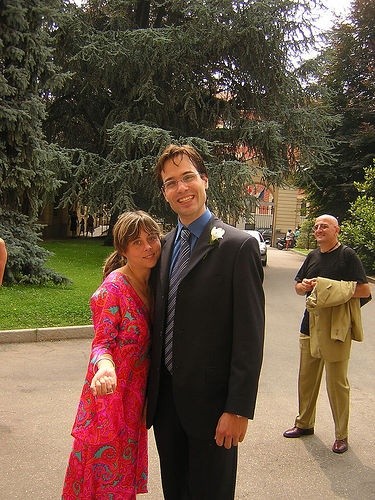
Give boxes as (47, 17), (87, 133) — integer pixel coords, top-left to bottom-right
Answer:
(276, 232), (297, 250)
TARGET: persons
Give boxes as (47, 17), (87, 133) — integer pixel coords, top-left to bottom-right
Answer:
(80, 218), (85, 237)
(284, 214), (371, 453)
(147, 144), (265, 500)
(0, 237), (8, 286)
(61, 211), (162, 500)
(68, 211), (79, 237)
(284, 226), (301, 250)
(86, 215), (94, 237)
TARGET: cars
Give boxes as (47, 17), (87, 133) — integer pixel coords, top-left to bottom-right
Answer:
(238, 230), (267, 266)
(258, 229), (272, 245)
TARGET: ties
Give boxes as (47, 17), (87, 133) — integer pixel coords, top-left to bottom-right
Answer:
(165, 229), (191, 376)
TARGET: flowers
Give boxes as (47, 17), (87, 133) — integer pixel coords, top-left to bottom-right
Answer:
(208, 226), (226, 246)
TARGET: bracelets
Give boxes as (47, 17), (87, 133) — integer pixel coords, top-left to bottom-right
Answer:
(235, 414), (243, 418)
(92, 357), (115, 375)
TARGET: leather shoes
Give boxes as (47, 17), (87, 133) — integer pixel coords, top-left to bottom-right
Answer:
(283, 426), (314, 438)
(332, 438), (348, 454)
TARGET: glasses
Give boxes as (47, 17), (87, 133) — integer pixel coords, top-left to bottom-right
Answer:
(312, 225), (329, 231)
(161, 172), (200, 192)
